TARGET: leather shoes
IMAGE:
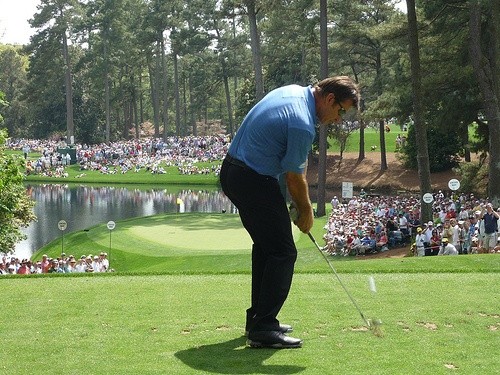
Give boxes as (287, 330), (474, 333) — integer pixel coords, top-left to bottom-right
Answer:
(244, 323), (291, 336)
(246, 329), (301, 348)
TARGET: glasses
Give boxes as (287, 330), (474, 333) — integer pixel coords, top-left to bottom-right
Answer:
(338, 102), (346, 117)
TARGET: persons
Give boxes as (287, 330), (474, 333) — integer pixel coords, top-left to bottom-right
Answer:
(24, 183), (238, 212)
(5, 133), (231, 178)
(220, 76), (360, 348)
(0, 252), (115, 274)
(331, 116), (415, 152)
(410, 190), (500, 257)
(320, 189), (421, 257)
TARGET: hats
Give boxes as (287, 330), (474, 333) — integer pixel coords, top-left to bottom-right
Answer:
(428, 221), (433, 225)
(441, 238), (448, 242)
(417, 227), (422, 232)
(436, 224), (442, 227)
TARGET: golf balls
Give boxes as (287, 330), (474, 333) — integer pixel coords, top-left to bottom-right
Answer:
(289, 207), (299, 219)
(370, 276), (377, 293)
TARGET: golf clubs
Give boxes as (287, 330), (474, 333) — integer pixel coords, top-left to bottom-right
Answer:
(307, 231), (382, 328)
